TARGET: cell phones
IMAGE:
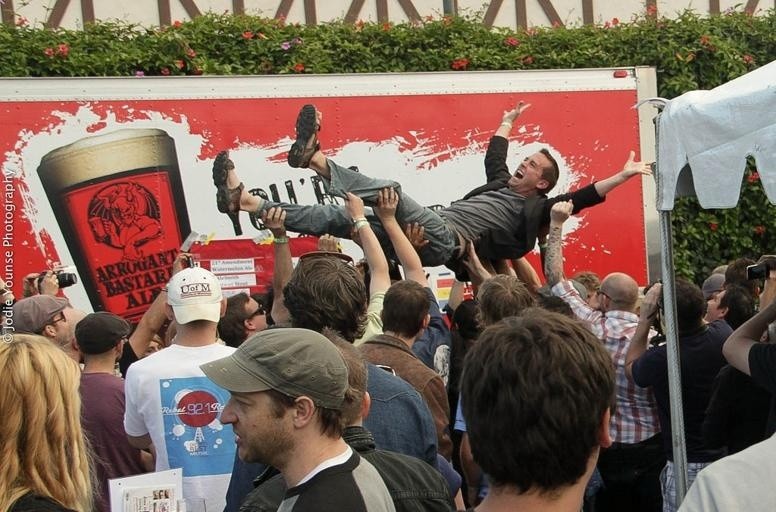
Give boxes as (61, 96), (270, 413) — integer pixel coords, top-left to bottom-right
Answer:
(746, 263), (770, 280)
(187, 257), (193, 268)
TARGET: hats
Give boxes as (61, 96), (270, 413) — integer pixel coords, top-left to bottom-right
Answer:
(200, 328), (349, 411)
(12, 294), (69, 335)
(75, 311), (131, 354)
(166, 266), (222, 326)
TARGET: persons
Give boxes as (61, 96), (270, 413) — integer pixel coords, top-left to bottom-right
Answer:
(213, 100), (653, 264)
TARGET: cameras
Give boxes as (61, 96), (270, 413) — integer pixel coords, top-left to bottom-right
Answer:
(38, 270), (77, 294)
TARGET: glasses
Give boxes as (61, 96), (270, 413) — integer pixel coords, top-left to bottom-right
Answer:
(296, 251), (355, 279)
(248, 303), (264, 320)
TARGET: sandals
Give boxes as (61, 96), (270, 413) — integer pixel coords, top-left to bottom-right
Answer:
(286, 104), (321, 168)
(213, 150), (244, 214)
